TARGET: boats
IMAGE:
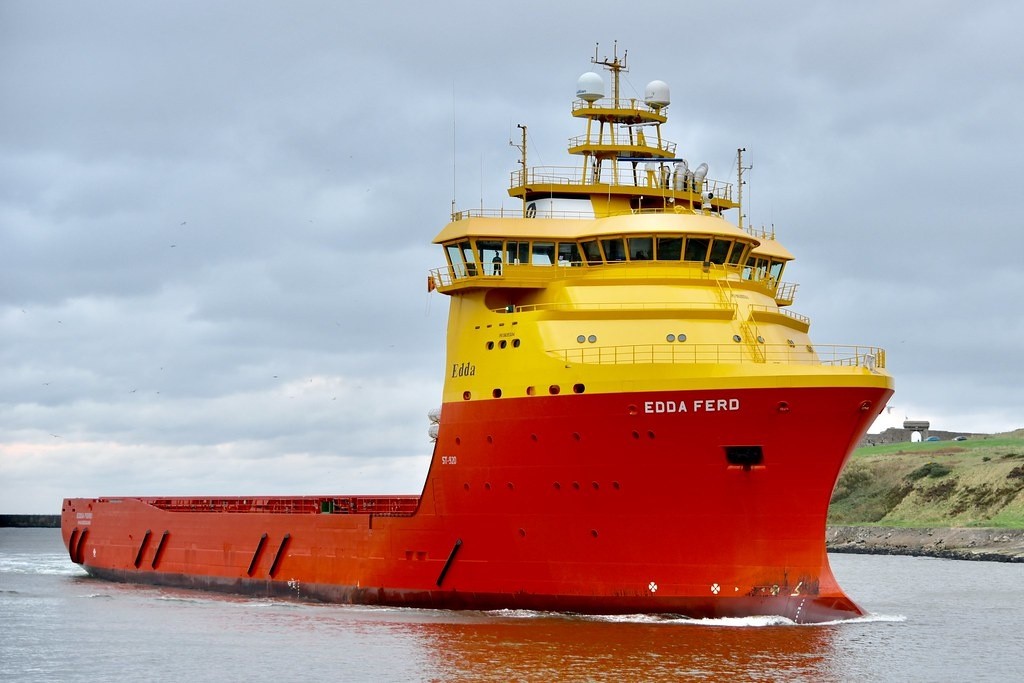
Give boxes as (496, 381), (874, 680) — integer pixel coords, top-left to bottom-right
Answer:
(61, 28), (898, 622)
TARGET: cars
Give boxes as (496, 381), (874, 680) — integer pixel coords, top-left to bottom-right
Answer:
(924, 435), (941, 442)
(954, 436), (968, 441)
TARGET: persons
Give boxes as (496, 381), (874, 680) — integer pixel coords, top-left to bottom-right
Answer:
(492, 252), (501, 276)
